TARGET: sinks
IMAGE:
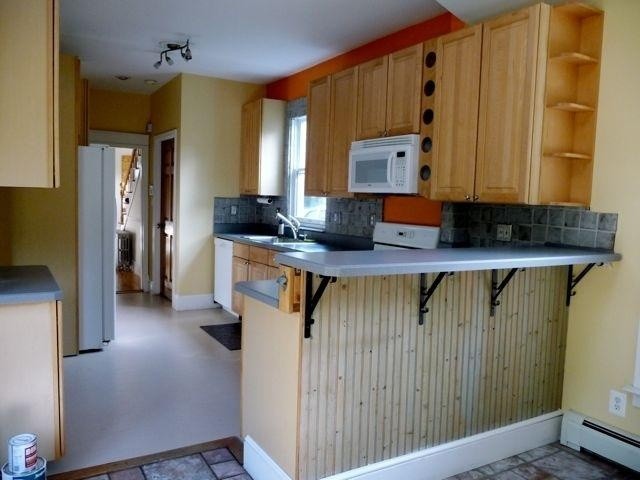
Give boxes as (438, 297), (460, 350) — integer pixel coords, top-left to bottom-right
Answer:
(241, 236), (304, 244)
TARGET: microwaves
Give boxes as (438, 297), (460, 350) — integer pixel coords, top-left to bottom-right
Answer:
(348, 134), (419, 193)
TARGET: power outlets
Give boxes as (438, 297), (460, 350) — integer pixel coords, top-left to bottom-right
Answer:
(497, 225), (511, 241)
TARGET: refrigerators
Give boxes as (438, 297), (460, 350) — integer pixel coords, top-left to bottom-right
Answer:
(79, 146), (116, 351)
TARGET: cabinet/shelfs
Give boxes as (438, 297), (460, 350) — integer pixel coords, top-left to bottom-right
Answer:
(356, 56), (387, 138)
(538, 0), (604, 207)
(1, 1), (60, 190)
(305, 66), (358, 198)
(248, 244), (267, 281)
(212, 236), (249, 319)
(241, 98), (285, 196)
(268, 249), (280, 280)
(386, 41), (423, 135)
(430, 2), (541, 205)
(0, 301), (66, 461)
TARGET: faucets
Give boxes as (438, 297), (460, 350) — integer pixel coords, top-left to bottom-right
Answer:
(274, 208), (300, 239)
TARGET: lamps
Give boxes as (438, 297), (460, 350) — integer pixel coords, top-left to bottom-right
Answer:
(153, 41), (192, 69)
